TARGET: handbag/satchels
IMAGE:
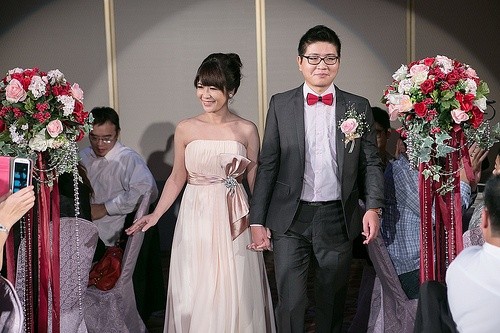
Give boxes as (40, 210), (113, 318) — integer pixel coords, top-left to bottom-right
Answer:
(86, 246), (122, 291)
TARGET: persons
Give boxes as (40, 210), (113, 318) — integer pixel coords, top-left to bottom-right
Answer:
(78, 106), (159, 247)
(247, 24), (387, 333)
(370, 106), (500, 333)
(123, 52), (272, 333)
(0, 184), (36, 333)
(50, 160), (107, 264)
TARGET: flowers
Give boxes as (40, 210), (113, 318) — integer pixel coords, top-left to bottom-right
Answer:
(0, 64), (95, 333)
(380, 54), (500, 283)
(337, 101), (374, 153)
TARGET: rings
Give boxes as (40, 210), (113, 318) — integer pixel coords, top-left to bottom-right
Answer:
(133, 219), (138, 224)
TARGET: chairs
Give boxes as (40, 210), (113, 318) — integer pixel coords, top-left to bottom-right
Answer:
(359, 199), (418, 333)
(84, 191), (154, 333)
(0, 217), (99, 333)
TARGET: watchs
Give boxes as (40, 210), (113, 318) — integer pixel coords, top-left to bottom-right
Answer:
(370, 207), (383, 218)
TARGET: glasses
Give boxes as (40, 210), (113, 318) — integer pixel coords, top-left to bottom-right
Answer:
(375, 128), (387, 138)
(300, 54), (339, 65)
(89, 133), (117, 144)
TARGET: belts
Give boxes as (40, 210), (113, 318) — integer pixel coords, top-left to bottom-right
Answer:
(299, 200), (341, 206)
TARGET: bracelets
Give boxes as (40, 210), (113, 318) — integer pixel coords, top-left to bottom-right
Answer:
(0, 224), (9, 235)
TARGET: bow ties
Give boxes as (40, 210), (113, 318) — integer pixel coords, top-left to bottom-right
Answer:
(307, 93), (333, 106)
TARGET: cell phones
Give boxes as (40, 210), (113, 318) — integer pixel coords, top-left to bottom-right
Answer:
(11, 158), (31, 194)
